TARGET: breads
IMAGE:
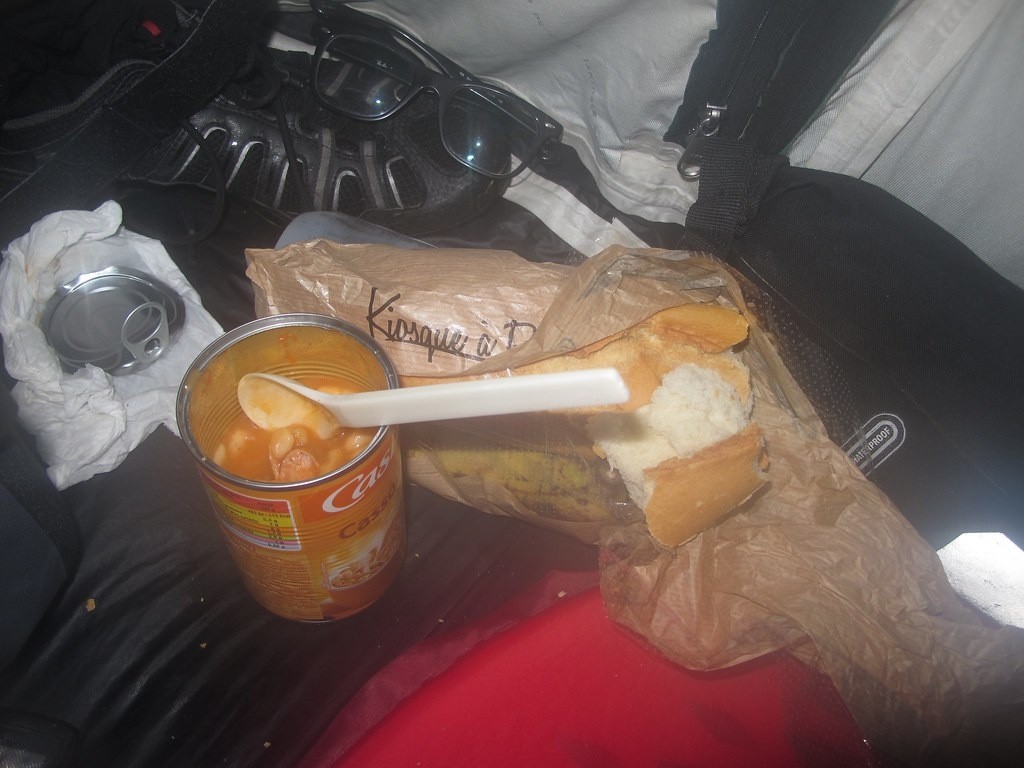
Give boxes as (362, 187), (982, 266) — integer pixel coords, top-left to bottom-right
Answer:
(397, 304), (771, 549)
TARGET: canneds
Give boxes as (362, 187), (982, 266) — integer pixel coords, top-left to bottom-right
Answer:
(175, 310), (405, 624)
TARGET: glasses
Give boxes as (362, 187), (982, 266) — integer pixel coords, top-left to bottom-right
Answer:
(309, 1), (562, 181)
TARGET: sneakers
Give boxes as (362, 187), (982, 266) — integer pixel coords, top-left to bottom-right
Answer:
(116, 46), (512, 236)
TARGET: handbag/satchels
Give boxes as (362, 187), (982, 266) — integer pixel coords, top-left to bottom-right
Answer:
(0, 0), (281, 235)
(685, 138), (1024, 632)
